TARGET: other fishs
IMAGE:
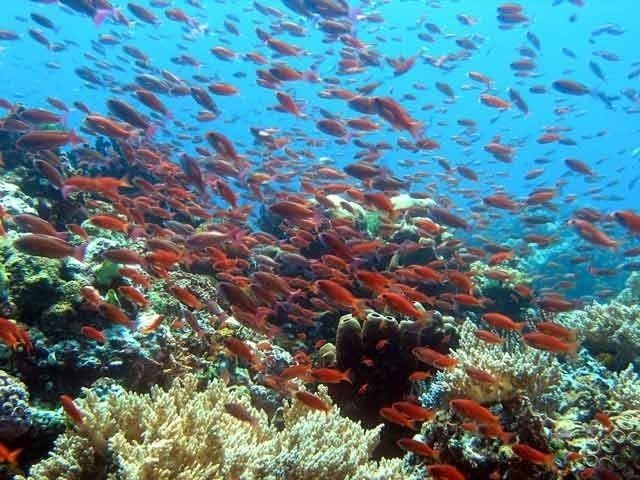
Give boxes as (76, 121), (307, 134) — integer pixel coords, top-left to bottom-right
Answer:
(0, 0), (640, 480)
